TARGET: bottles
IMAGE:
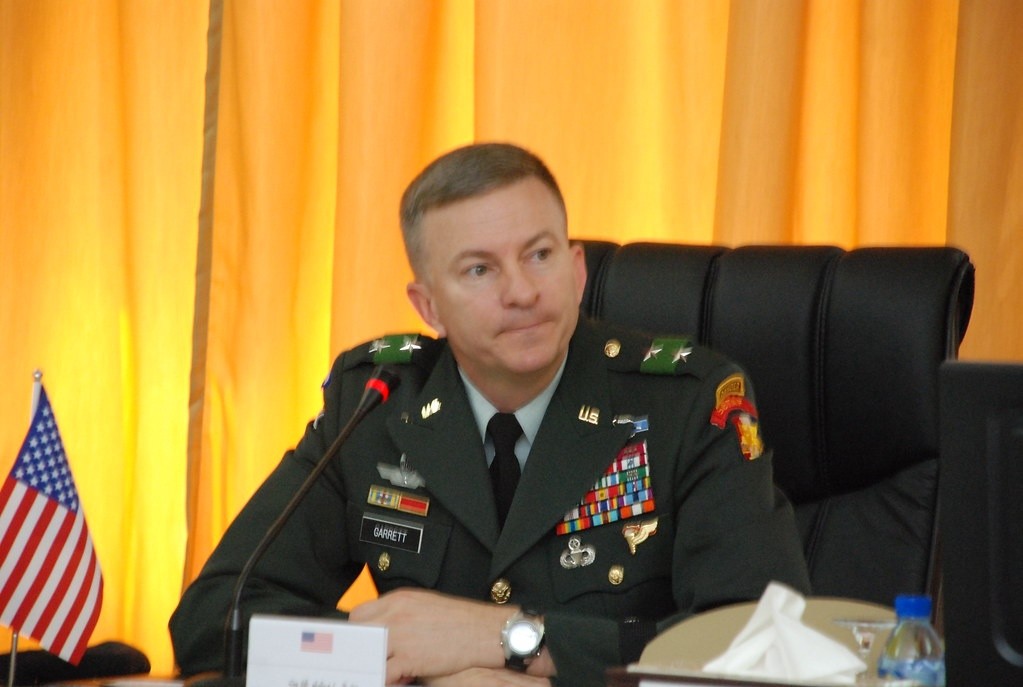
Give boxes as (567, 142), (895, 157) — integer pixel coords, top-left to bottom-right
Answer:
(877, 595), (946, 687)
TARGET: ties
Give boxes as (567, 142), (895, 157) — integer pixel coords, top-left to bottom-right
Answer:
(487, 412), (524, 531)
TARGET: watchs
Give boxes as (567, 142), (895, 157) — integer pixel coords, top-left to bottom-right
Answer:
(499, 603), (548, 674)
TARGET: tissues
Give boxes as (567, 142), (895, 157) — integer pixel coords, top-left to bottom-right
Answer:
(604, 579), (899, 686)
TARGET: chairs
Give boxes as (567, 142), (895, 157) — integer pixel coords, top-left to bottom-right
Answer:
(568, 239), (975, 623)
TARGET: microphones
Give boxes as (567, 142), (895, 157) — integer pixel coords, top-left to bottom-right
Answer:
(186, 368), (404, 687)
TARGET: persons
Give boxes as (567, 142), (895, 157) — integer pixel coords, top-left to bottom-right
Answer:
(168, 143), (813, 687)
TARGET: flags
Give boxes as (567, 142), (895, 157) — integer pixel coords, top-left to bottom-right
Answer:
(0, 382), (104, 668)
